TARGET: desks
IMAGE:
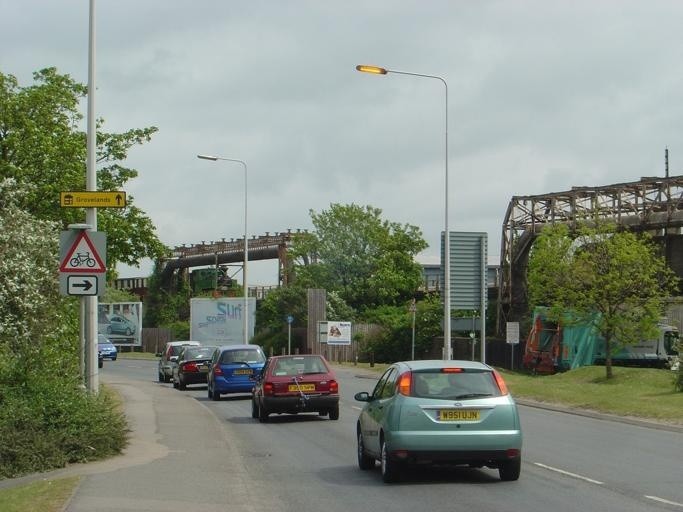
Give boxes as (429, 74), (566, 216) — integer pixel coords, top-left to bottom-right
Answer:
(354, 64), (452, 346)
(197, 153), (248, 345)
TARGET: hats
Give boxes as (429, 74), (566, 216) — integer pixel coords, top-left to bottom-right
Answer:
(154, 340), (339, 420)
(108, 315), (135, 336)
(96, 334), (116, 361)
(353, 360), (522, 479)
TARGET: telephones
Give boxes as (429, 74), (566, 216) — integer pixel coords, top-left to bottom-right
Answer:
(59, 191), (126, 208)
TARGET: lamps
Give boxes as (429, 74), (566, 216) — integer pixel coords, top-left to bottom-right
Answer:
(415, 374), (484, 396)
